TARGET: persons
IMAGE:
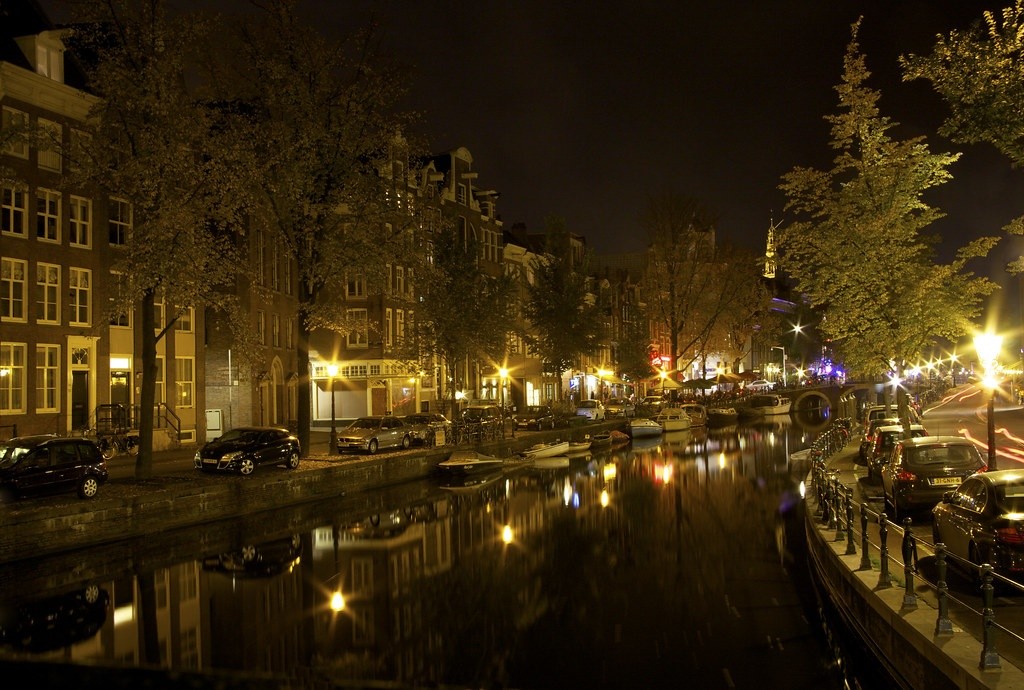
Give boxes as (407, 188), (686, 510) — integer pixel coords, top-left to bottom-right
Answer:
(590, 385), (611, 406)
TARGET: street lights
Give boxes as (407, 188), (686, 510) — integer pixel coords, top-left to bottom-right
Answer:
(914, 365), (920, 402)
(660, 371), (668, 395)
(937, 358), (943, 384)
(950, 354), (957, 388)
(499, 367), (508, 405)
(927, 363), (933, 403)
(973, 334), (1003, 472)
(328, 363), (338, 455)
(598, 369), (606, 404)
(770, 346), (786, 387)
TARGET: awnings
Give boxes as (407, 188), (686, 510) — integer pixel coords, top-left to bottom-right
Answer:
(591, 374), (633, 385)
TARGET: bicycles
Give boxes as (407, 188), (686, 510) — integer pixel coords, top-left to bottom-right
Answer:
(834, 416), (854, 452)
(96, 422), (140, 460)
(813, 450), (853, 526)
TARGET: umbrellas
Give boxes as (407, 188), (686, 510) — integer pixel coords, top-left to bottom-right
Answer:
(652, 371), (757, 401)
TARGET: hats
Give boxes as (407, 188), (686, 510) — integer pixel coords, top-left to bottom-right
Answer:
(905, 393), (914, 402)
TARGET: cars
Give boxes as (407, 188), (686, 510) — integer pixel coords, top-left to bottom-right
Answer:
(745, 379), (777, 391)
(3, 586), (114, 654)
(512, 405), (556, 431)
(575, 396), (668, 421)
(858, 404), (1024, 590)
(0, 433), (109, 501)
(199, 504), (417, 577)
(338, 404), (505, 455)
(193, 425), (302, 476)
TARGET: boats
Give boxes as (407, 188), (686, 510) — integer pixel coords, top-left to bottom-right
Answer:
(658, 394), (793, 432)
(437, 448), (505, 476)
(517, 419), (663, 459)
